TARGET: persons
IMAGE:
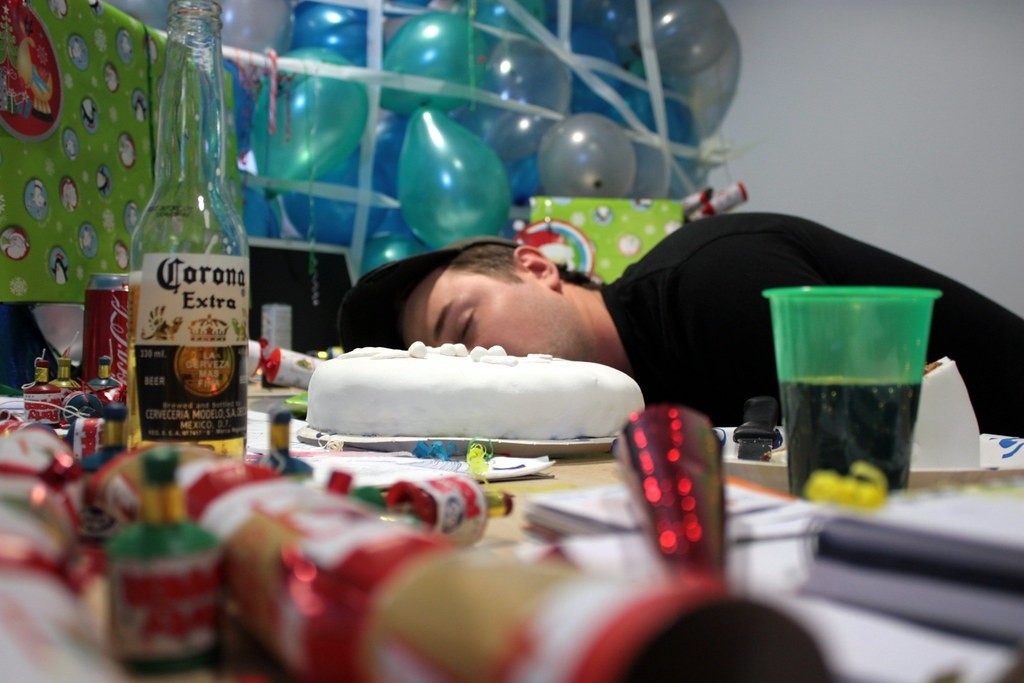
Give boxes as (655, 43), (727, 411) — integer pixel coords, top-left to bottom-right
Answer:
(335, 213), (1023, 437)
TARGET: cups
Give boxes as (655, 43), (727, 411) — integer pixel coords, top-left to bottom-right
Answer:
(762, 288), (941, 500)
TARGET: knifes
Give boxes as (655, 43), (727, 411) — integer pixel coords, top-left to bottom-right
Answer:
(733, 398), (782, 462)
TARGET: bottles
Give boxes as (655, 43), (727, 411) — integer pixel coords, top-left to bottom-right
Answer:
(85, 356), (121, 403)
(256, 408), (315, 483)
(80, 404), (129, 547)
(390, 473), (512, 545)
(62, 391), (102, 425)
(50, 357), (82, 401)
(265, 347), (325, 389)
(24, 360), (61, 429)
(109, 449), (221, 674)
(127, 0), (250, 458)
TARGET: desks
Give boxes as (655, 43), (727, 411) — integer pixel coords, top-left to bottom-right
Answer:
(141, 434), (801, 549)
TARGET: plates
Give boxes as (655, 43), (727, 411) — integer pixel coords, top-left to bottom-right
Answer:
(714, 427), (1024, 489)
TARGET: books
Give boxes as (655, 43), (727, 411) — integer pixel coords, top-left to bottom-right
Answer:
(526, 476), (813, 542)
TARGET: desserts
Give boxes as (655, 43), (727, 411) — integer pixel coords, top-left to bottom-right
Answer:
(911, 355), (981, 471)
(305, 341), (644, 441)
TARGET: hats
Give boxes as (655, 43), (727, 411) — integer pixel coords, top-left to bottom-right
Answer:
(337, 237), (565, 354)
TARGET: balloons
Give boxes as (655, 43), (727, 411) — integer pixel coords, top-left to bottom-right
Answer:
(112, 1), (740, 277)
